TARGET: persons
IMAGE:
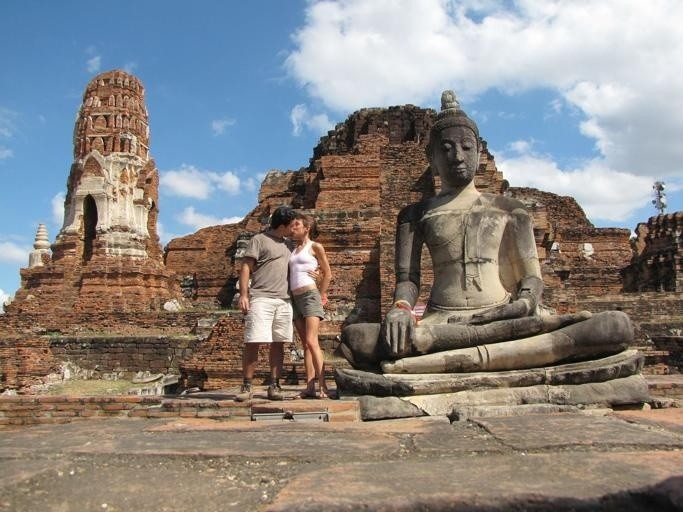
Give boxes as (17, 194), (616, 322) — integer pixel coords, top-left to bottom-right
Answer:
(341, 90), (635, 374)
(288, 212), (332, 399)
(236, 206), (322, 401)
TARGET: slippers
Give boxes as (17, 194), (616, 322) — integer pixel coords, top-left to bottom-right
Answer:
(295, 391), (317, 399)
(319, 388), (329, 399)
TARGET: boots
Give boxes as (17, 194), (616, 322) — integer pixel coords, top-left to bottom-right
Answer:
(236, 384), (253, 401)
(268, 383), (284, 400)
